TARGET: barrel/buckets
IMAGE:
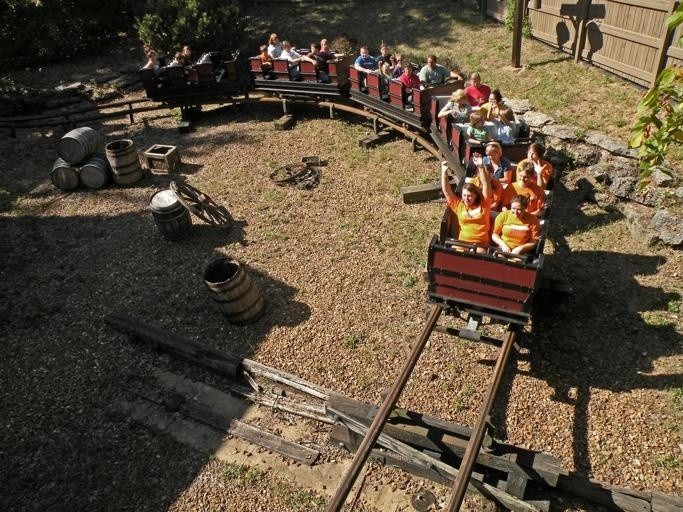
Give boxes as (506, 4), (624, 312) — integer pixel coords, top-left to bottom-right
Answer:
(51, 156), (81, 191)
(149, 188), (193, 241)
(105, 138), (142, 185)
(79, 152), (109, 189)
(202, 257), (265, 326)
(56, 126), (100, 165)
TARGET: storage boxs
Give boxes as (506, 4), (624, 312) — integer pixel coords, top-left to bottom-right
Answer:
(142, 144), (180, 175)
